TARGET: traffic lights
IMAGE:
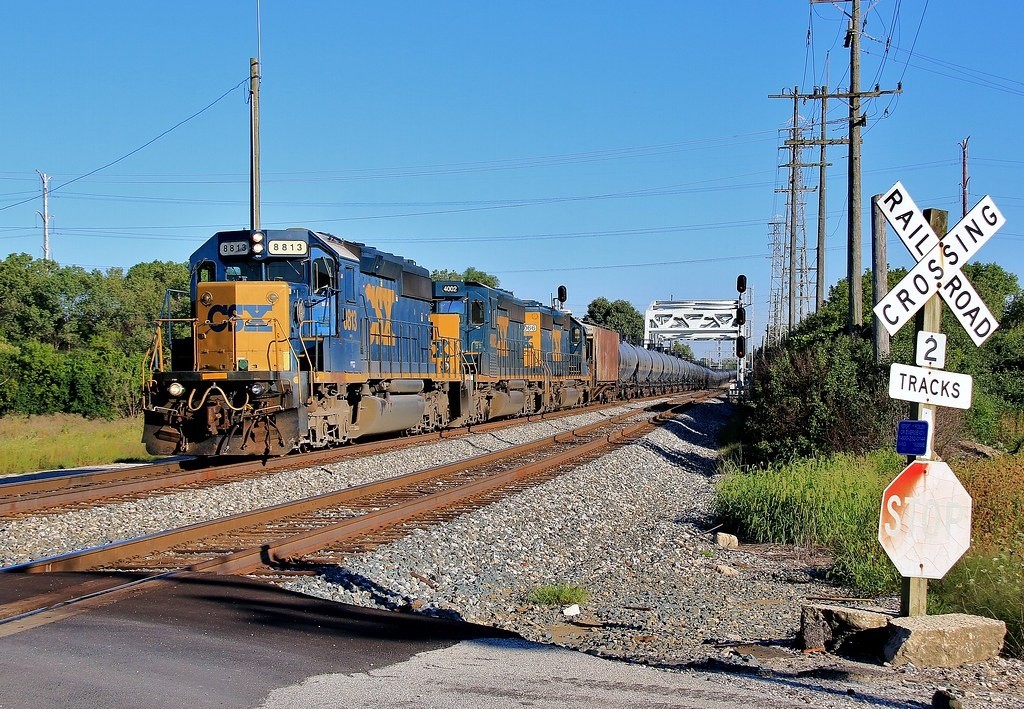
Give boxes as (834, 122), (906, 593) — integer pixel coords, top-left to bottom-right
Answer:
(558, 286), (566, 303)
(737, 275), (747, 293)
(737, 307), (745, 325)
(735, 336), (745, 358)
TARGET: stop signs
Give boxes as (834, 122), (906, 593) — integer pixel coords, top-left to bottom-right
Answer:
(878, 460), (973, 579)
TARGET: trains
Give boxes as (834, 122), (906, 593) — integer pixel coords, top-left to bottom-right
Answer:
(141, 227), (739, 465)
